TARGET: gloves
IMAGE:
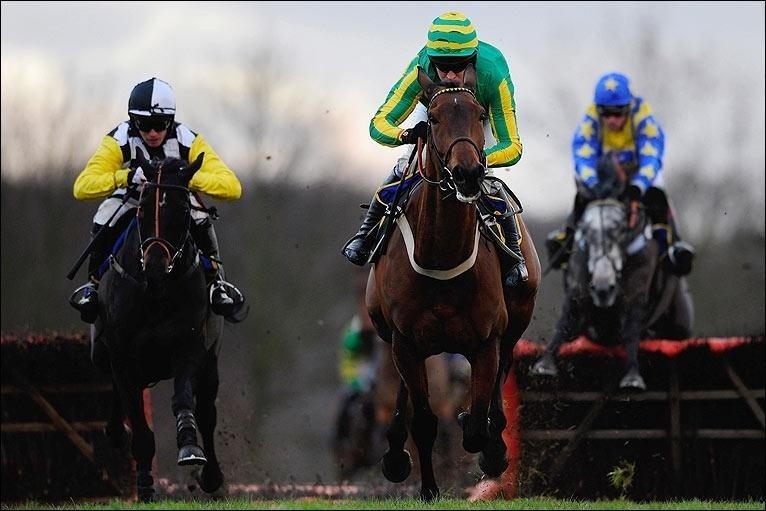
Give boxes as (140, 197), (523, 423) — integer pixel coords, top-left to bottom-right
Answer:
(401, 121), (427, 144)
(591, 184), (612, 200)
(128, 167), (147, 187)
(619, 184), (641, 212)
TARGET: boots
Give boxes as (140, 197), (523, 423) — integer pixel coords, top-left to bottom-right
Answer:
(546, 216), (573, 263)
(186, 216), (234, 304)
(77, 232), (105, 323)
(476, 189), (528, 287)
(345, 165), (400, 265)
(651, 222), (696, 276)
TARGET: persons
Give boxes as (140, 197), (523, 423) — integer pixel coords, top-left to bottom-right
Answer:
(336, 282), (387, 487)
(346, 13), (523, 289)
(73, 77), (242, 308)
(546, 73), (694, 278)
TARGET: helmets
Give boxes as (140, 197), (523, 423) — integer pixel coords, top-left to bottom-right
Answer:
(427, 11), (478, 57)
(128, 78), (176, 116)
(595, 74), (633, 105)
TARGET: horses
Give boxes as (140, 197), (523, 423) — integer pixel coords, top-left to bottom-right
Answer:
(527, 175), (695, 391)
(365, 63), (542, 505)
(96, 146), (226, 504)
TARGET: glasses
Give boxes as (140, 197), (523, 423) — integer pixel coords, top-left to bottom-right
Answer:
(132, 117), (171, 132)
(427, 52), (477, 73)
(597, 105), (628, 118)
